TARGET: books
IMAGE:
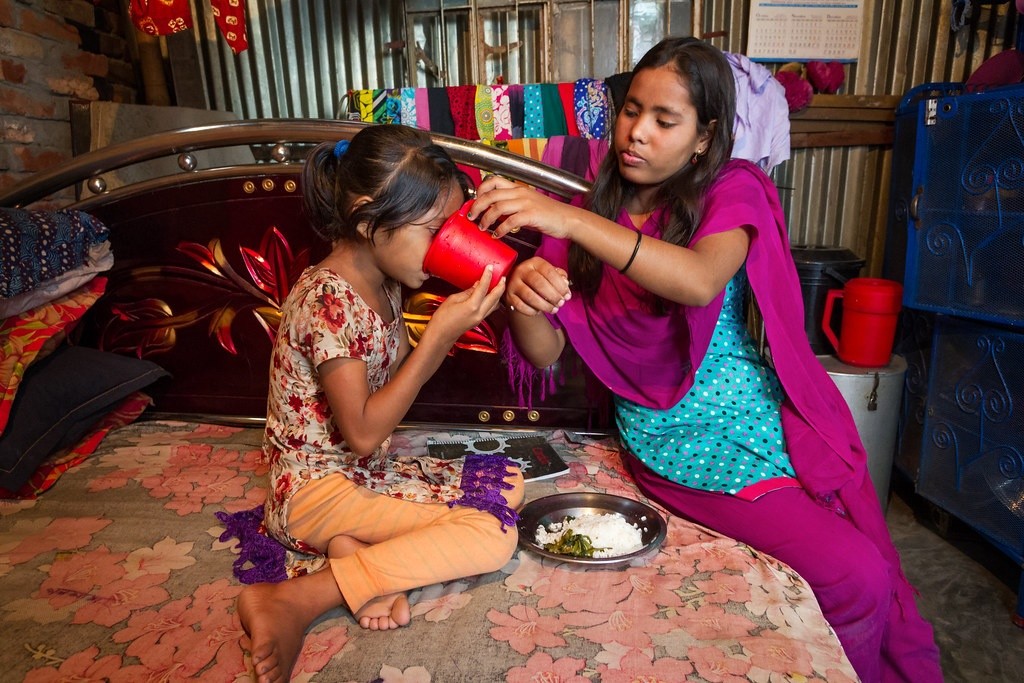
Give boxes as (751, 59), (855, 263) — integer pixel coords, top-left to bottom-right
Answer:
(427, 436), (570, 483)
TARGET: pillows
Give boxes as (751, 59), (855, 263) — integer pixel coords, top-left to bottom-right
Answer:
(0, 204), (114, 304)
(0, 393), (156, 500)
(0, 347), (175, 496)
(0, 249), (116, 319)
(0, 276), (112, 429)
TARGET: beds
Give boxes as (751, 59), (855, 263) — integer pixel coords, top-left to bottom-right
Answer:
(0, 119), (867, 683)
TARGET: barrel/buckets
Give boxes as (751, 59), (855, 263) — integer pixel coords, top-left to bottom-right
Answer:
(790, 245), (866, 354)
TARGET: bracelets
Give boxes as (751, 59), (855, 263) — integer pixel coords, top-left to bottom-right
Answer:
(620, 231), (641, 275)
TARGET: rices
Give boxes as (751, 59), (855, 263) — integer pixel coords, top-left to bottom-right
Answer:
(536, 513), (648, 559)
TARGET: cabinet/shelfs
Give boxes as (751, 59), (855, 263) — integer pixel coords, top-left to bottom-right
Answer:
(879, 79), (1024, 627)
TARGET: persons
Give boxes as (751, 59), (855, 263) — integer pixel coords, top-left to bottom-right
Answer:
(238, 124), (526, 683)
(467, 36), (944, 683)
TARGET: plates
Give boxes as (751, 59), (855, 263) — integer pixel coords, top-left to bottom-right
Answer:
(516, 491), (667, 564)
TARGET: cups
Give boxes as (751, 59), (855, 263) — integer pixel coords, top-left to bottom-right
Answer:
(422, 199), (517, 297)
(823, 277), (902, 368)
